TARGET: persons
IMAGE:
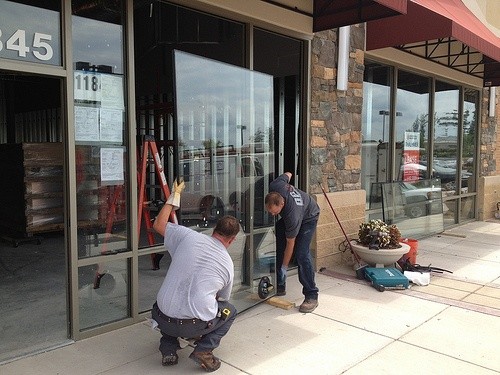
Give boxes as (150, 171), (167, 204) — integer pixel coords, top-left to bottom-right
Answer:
(151, 176), (238, 373)
(265, 172), (319, 313)
(181, 172), (279, 293)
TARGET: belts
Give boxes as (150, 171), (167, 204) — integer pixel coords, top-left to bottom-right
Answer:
(153, 302), (202, 326)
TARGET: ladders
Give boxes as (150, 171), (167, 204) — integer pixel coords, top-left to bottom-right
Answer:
(94, 135), (179, 290)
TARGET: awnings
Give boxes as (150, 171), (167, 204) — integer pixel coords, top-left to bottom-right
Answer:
(366, 0), (500, 87)
(313, 0), (407, 33)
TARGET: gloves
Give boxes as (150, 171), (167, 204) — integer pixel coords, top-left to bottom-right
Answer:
(276, 264), (288, 286)
(163, 178), (187, 210)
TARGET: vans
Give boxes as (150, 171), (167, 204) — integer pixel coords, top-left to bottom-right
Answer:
(155, 154), (264, 221)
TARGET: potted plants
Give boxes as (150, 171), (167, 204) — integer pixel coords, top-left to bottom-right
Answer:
(349, 218), (410, 268)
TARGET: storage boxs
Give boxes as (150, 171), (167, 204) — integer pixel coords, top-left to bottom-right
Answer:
(364, 268), (409, 292)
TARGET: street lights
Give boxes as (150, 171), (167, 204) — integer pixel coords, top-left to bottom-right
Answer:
(379, 110), (390, 142)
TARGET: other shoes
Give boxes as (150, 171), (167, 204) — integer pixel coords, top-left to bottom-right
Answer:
(188, 349), (222, 372)
(161, 350), (179, 366)
(276, 286), (287, 296)
(298, 297), (318, 313)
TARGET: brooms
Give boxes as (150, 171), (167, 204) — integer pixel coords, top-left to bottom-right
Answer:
(317, 181), (370, 280)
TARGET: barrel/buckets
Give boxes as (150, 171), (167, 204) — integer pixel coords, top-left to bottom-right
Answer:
(398, 238), (418, 270)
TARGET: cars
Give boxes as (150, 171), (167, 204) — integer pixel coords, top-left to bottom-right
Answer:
(400, 158), (472, 218)
